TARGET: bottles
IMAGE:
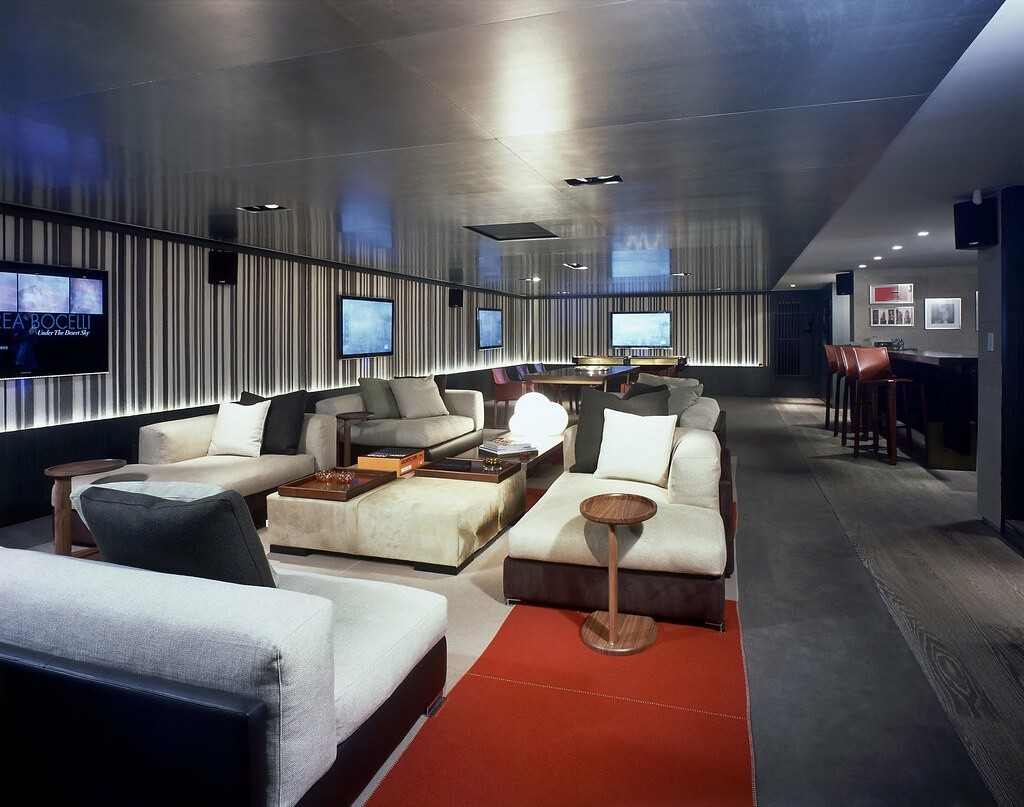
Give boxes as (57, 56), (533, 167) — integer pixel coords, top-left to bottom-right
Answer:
(873, 309), (910, 325)
(892, 338), (904, 351)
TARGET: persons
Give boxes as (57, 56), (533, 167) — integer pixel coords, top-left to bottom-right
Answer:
(934, 308), (951, 324)
(873, 310), (911, 324)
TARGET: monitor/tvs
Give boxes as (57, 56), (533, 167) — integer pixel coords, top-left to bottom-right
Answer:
(477, 308), (503, 351)
(337, 295), (394, 360)
(0, 259), (109, 380)
(610, 312), (674, 350)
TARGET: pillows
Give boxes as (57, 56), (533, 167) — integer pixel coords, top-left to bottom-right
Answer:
(358, 374), (449, 420)
(207, 390), (308, 457)
(569, 373), (704, 489)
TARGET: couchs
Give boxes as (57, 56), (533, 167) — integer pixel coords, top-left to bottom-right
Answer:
(503, 396), (734, 631)
(0, 544), (449, 807)
(52, 414), (337, 548)
(316, 389), (484, 462)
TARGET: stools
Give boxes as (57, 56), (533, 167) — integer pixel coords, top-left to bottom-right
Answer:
(824, 344), (913, 465)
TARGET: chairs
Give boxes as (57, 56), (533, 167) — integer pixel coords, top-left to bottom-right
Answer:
(621, 365), (676, 398)
(492, 363), (557, 429)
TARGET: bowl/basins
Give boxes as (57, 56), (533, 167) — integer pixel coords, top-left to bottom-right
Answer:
(482, 457), (503, 471)
(315, 468), (335, 482)
(337, 469), (355, 483)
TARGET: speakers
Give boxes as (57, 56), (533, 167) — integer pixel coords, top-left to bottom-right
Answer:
(954, 197), (999, 251)
(836, 272), (853, 295)
(448, 289), (463, 308)
(208, 251), (238, 285)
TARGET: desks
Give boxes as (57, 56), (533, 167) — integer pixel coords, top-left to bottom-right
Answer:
(524, 366), (640, 415)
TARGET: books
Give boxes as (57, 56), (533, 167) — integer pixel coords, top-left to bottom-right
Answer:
(479, 444), (538, 456)
(483, 437), (531, 452)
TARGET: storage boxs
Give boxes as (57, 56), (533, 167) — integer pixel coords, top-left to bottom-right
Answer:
(358, 448), (425, 477)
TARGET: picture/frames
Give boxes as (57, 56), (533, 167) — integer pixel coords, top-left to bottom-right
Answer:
(869, 283), (914, 304)
(869, 307), (915, 326)
(924, 297), (962, 330)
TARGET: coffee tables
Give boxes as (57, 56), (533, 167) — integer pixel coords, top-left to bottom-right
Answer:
(266, 469), (526, 576)
(452, 430), (564, 494)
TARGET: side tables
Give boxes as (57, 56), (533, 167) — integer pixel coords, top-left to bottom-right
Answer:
(580, 493), (658, 656)
(336, 411), (375, 467)
(44, 458), (127, 556)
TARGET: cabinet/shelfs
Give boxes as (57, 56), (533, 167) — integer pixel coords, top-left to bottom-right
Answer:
(572, 356), (690, 378)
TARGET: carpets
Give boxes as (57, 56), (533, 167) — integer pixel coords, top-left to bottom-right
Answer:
(27, 488), (754, 807)
